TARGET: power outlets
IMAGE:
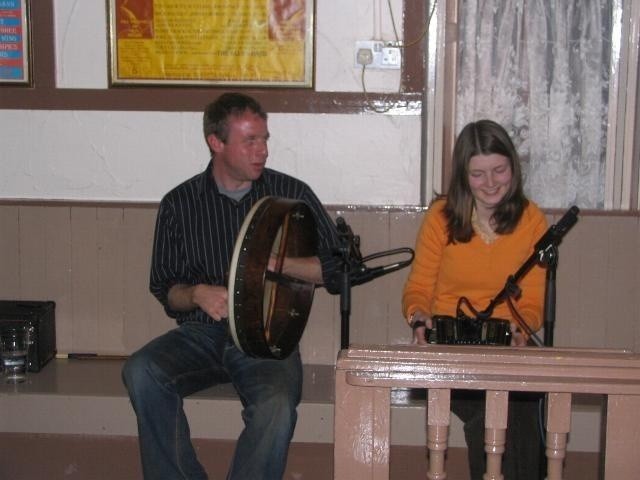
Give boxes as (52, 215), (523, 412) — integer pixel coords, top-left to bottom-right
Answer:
(355, 40), (403, 70)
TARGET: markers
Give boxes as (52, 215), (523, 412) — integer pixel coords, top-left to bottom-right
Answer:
(55, 354), (97, 359)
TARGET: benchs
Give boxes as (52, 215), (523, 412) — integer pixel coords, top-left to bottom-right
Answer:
(0, 197), (640, 455)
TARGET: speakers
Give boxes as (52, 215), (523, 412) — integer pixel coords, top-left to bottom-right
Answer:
(0, 301), (56, 373)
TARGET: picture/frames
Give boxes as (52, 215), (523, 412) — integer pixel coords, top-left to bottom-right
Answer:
(105, 1), (316, 89)
(0, 1), (35, 87)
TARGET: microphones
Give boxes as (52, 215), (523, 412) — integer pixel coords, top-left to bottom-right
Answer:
(325, 261), (408, 294)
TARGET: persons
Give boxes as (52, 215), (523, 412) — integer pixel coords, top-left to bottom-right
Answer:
(121, 91), (359, 480)
(401, 118), (554, 480)
